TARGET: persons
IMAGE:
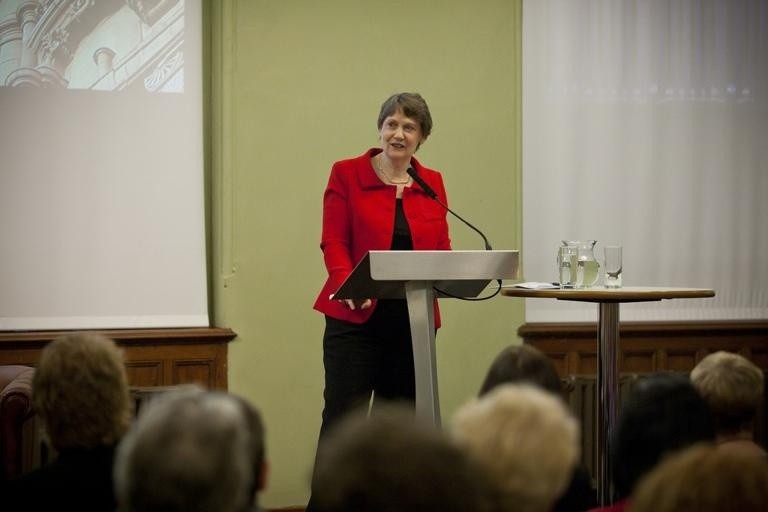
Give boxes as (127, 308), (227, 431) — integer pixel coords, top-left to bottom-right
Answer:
(308, 92), (452, 512)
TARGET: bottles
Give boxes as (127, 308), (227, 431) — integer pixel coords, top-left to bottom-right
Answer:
(562, 239), (598, 286)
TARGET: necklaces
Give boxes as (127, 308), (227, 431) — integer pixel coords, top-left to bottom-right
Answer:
(376, 157), (412, 184)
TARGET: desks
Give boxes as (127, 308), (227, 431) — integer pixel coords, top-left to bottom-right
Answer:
(497, 283), (714, 511)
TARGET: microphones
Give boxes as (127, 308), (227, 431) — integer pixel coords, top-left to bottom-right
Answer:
(407, 168), (492, 250)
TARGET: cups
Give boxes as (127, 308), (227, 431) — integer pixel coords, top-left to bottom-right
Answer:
(604, 245), (623, 290)
(558, 246), (578, 289)
